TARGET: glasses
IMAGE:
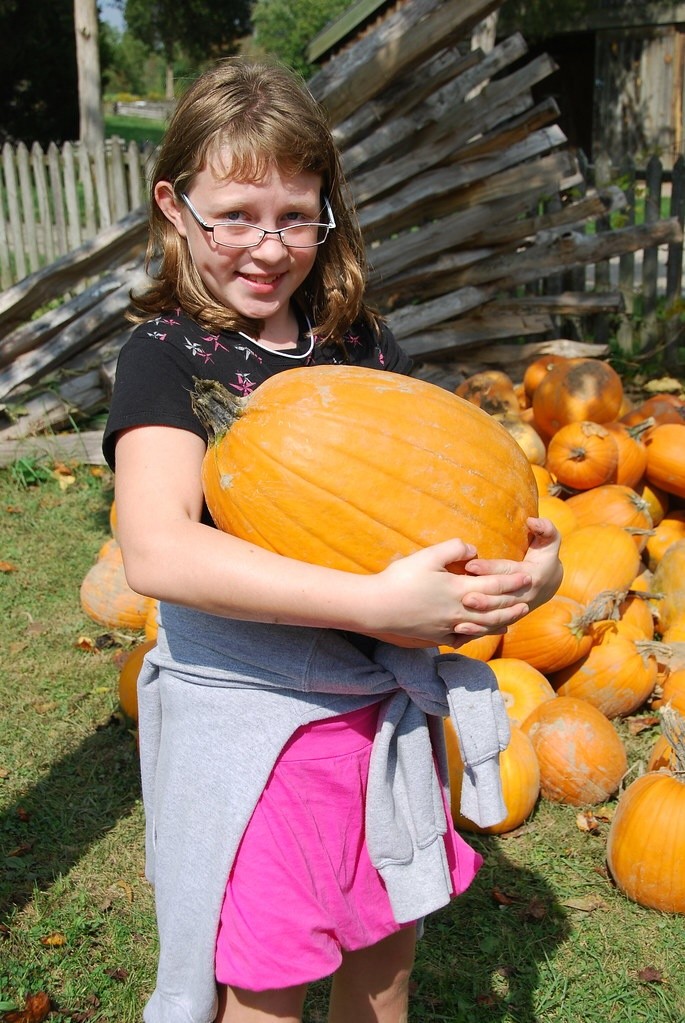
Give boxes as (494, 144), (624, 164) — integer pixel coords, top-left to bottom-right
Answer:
(175, 191), (338, 249)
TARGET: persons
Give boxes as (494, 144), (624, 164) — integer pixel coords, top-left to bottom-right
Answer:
(101, 57), (561, 1023)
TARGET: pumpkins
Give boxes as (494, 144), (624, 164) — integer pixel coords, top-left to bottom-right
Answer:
(78, 357), (684, 918)
